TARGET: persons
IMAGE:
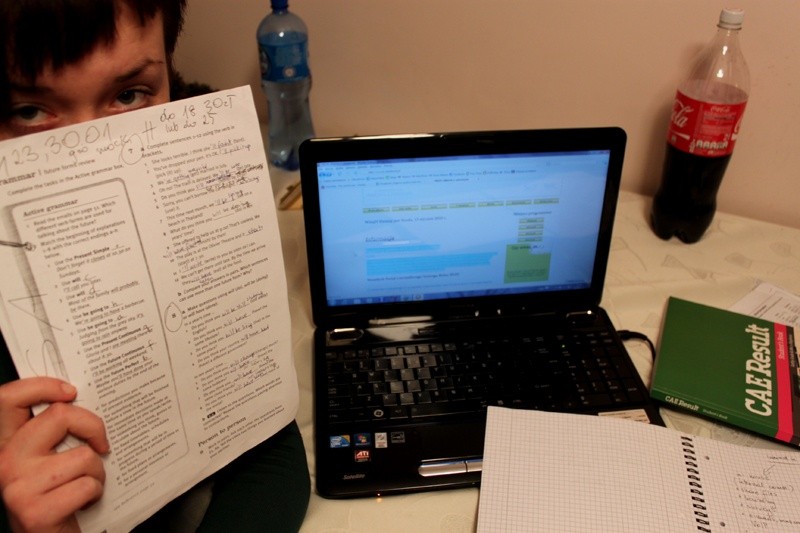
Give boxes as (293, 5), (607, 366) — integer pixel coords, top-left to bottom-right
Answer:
(0, 0), (311, 533)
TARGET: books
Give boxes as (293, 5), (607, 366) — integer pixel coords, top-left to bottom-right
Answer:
(476, 406), (800, 533)
(649, 295), (800, 448)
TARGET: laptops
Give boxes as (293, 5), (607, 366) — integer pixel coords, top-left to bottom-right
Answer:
(299, 126), (669, 501)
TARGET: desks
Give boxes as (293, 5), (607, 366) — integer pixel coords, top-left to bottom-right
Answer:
(176, 170), (800, 533)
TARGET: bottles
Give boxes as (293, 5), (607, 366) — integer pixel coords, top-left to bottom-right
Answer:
(649, 7), (752, 244)
(256, 0), (315, 171)
